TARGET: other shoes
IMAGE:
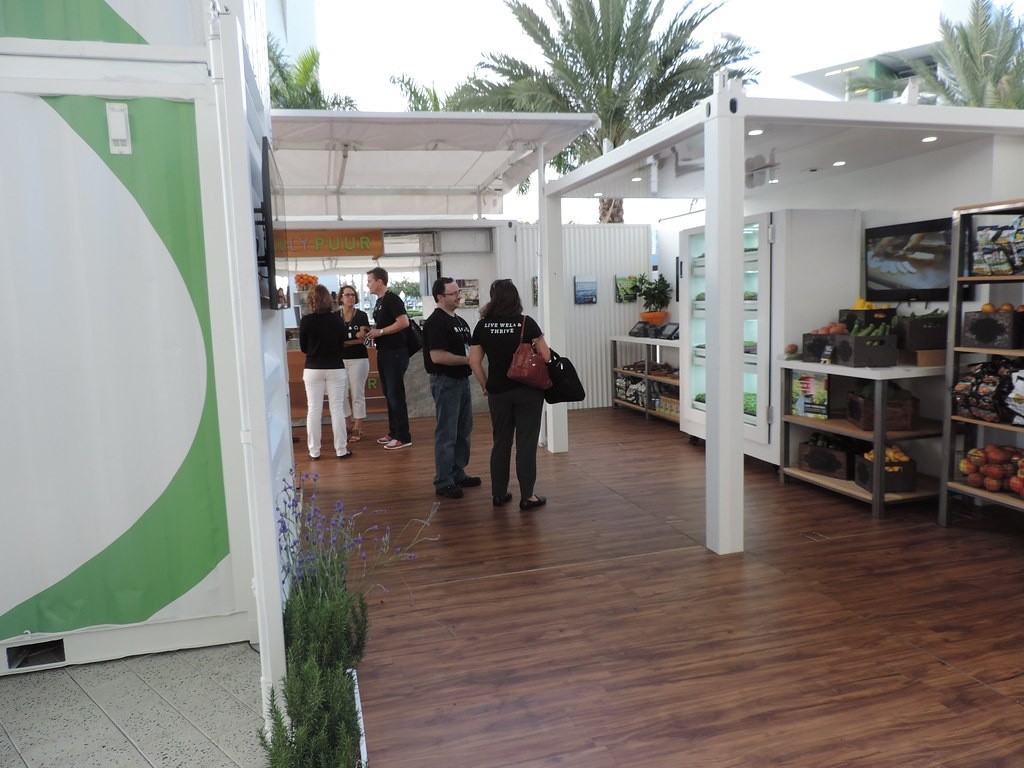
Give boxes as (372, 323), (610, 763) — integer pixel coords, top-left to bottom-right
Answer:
(492, 491), (513, 506)
(309, 453), (320, 460)
(337, 451), (352, 459)
(383, 439), (413, 450)
(377, 435), (394, 445)
(519, 495), (546, 509)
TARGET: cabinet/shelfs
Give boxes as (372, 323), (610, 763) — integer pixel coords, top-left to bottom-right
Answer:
(606, 335), (680, 430)
(779, 197), (1024, 528)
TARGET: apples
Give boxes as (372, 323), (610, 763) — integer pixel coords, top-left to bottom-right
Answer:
(810, 322), (849, 335)
(958, 443), (1024, 501)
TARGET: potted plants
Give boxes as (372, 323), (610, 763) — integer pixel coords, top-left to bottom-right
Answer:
(638, 272), (674, 327)
(250, 657), (368, 768)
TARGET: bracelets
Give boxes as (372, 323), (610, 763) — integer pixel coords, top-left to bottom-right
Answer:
(379, 329), (383, 335)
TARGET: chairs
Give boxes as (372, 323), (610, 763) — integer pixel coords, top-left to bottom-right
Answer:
(838, 308), (896, 332)
(803, 333), (833, 363)
(898, 313), (949, 351)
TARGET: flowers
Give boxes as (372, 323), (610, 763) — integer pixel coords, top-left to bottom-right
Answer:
(272, 459), (441, 671)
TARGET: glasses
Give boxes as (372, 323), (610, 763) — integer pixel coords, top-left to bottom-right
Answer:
(342, 293), (356, 297)
(441, 289), (463, 296)
(492, 279), (512, 289)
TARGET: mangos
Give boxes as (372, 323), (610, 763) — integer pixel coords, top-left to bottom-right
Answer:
(785, 344), (799, 354)
(863, 443), (910, 471)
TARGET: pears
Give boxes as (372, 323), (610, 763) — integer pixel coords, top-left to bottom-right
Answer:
(981, 302), (1024, 312)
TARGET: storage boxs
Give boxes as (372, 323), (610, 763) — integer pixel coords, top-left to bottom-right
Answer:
(798, 442), (857, 481)
(834, 335), (896, 368)
(845, 393), (918, 432)
(854, 453), (918, 496)
(962, 312), (1023, 350)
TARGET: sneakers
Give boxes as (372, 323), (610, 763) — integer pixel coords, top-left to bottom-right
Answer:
(435, 484), (464, 497)
(455, 475), (481, 487)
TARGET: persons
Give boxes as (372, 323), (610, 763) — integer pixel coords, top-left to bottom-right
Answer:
(331, 286), (370, 442)
(422, 276), (482, 498)
(299, 284), (352, 460)
(467, 280), (551, 510)
(363, 267), (413, 450)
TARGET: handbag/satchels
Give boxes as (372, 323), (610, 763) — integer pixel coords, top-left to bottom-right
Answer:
(406, 319), (427, 358)
(506, 315), (554, 391)
(545, 348), (586, 404)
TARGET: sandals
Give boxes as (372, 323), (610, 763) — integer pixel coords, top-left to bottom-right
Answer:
(346, 424), (364, 442)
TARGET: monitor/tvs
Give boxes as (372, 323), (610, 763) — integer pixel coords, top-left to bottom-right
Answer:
(865, 215), (974, 301)
(661, 323), (679, 337)
(253, 137), (291, 311)
(629, 321), (648, 336)
(418, 260), (441, 297)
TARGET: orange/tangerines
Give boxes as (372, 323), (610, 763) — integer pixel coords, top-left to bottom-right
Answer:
(295, 274), (319, 287)
